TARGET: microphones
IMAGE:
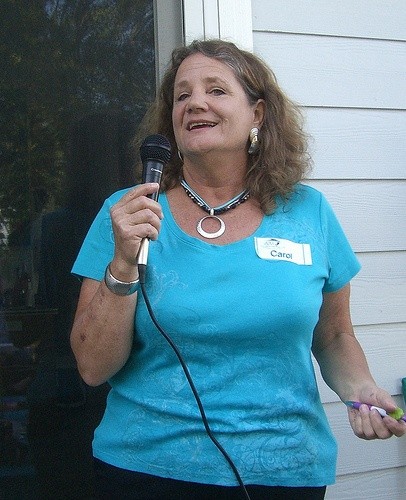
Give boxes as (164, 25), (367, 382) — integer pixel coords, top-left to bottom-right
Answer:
(136, 134), (173, 285)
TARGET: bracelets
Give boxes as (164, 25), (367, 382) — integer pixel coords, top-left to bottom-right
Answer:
(103, 263), (142, 296)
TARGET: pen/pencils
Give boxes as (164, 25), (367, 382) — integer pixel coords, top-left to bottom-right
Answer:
(345, 399), (406, 423)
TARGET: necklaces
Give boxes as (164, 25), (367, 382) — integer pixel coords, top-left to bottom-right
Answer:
(177, 175), (254, 240)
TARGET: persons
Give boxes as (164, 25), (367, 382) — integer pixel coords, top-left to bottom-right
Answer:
(69, 39), (406, 499)
